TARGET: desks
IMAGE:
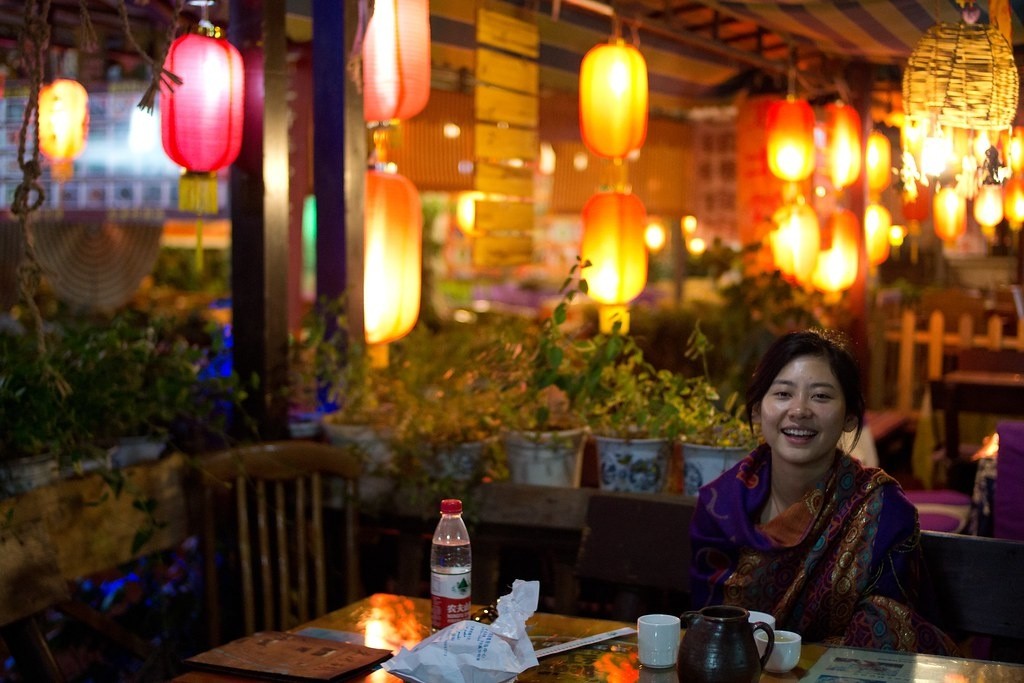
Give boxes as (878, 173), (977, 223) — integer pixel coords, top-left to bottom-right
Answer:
(929, 369), (1024, 490)
(165, 592), (1024, 683)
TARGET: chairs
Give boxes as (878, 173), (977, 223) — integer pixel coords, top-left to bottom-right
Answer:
(182, 436), (371, 651)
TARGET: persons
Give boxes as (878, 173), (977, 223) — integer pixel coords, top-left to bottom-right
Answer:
(689, 332), (965, 660)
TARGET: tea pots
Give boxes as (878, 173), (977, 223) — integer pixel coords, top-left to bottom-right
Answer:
(678, 604), (775, 683)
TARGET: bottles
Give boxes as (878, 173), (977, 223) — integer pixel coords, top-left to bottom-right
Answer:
(980, 457), (997, 505)
(431, 500), (472, 634)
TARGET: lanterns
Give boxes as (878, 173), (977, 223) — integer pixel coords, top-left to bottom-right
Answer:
(765, 95), (1024, 304)
(360, 0), (432, 145)
(582, 182), (649, 336)
(38, 79), (89, 180)
(160, 27), (245, 213)
(579, 37), (649, 163)
(361, 164), (422, 367)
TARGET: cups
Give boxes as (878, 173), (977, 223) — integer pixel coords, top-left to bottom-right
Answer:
(745, 611), (802, 674)
(637, 613), (680, 669)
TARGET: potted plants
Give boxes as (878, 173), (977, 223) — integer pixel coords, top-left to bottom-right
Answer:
(0, 256), (760, 498)
